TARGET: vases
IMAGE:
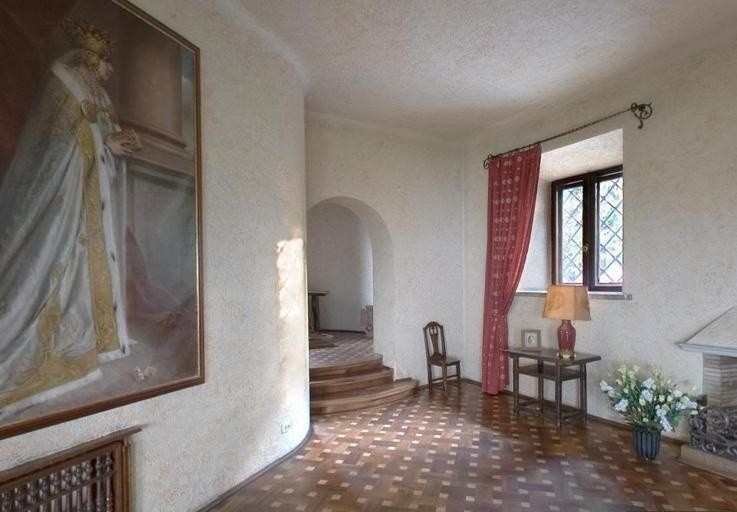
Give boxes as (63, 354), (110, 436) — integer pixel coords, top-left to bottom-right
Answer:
(632, 428), (660, 464)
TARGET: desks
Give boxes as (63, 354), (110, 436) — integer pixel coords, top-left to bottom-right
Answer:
(500, 347), (601, 430)
(308, 291), (328, 332)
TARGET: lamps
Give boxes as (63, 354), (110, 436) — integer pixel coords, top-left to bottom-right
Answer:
(543, 283), (591, 360)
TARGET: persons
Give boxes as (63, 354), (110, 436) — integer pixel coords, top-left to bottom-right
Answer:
(0, 18), (134, 421)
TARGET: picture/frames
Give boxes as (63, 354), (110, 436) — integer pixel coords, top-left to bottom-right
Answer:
(0, 0), (205, 440)
(521, 329), (541, 352)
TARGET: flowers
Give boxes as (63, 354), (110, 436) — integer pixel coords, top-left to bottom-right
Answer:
(599, 364), (705, 432)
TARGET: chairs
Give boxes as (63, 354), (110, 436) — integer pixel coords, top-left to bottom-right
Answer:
(423, 322), (461, 398)
